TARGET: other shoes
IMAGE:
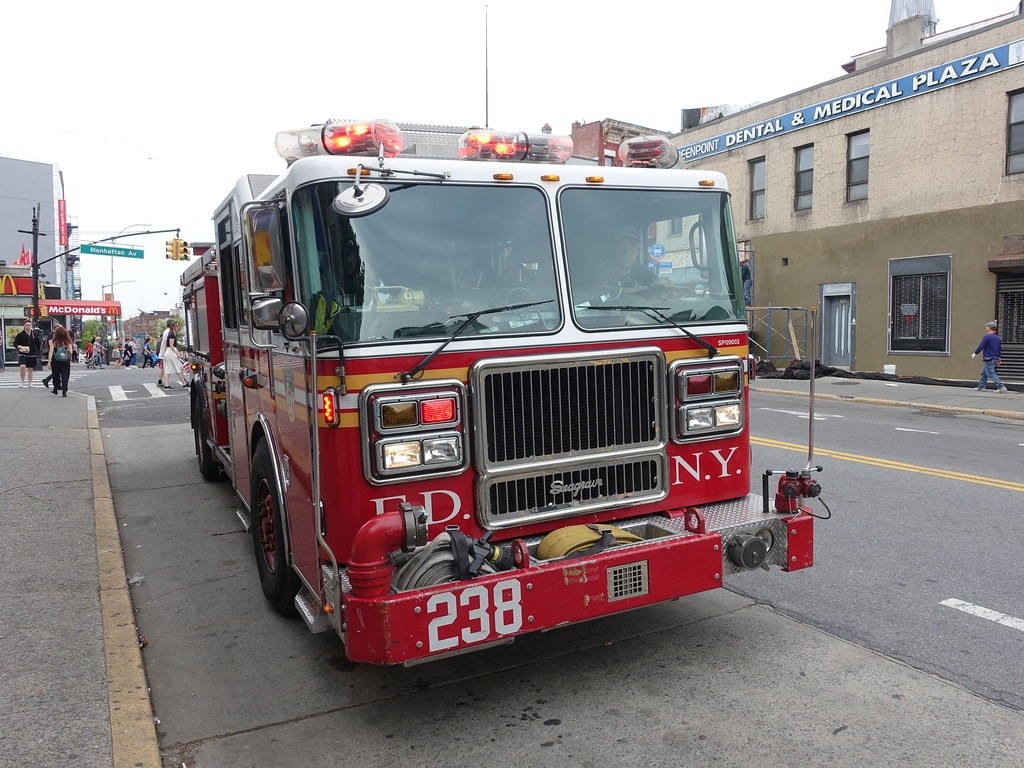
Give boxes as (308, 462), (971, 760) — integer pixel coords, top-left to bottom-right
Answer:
(28, 384), (35, 388)
(51, 390), (57, 394)
(974, 387), (986, 391)
(164, 385), (173, 390)
(183, 384), (190, 389)
(19, 381), (24, 387)
(994, 387), (1007, 392)
(42, 379), (49, 388)
(157, 380), (163, 387)
(63, 393), (66, 396)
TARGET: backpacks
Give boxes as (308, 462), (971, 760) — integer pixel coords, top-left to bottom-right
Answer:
(54, 344), (68, 361)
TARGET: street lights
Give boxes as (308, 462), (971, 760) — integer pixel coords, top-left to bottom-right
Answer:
(102, 280), (135, 301)
(111, 223), (152, 301)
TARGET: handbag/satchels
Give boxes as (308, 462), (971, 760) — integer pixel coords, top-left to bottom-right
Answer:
(19, 346), (30, 354)
(142, 345), (149, 356)
(111, 349), (120, 358)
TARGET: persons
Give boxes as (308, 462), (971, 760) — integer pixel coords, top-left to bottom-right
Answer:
(85, 320), (190, 390)
(42, 324), (78, 397)
(971, 322), (1007, 393)
(13, 320), (41, 388)
(574, 223), (673, 293)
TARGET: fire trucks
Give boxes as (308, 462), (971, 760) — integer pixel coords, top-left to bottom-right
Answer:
(178, 5), (828, 669)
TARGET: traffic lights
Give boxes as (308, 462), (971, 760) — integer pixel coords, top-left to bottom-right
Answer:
(165, 240), (178, 260)
(178, 240), (190, 261)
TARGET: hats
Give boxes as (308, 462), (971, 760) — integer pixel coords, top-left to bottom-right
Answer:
(616, 226), (642, 244)
(984, 322), (997, 329)
(740, 259), (743, 262)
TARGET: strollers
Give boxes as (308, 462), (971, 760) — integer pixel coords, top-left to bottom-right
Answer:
(85, 353), (101, 369)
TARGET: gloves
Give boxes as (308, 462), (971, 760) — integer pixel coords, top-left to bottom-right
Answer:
(972, 353), (976, 360)
(648, 278), (674, 286)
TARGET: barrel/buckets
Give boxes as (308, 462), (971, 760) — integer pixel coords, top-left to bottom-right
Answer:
(884, 365), (896, 375)
